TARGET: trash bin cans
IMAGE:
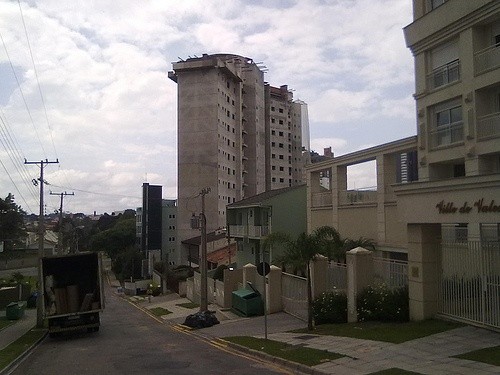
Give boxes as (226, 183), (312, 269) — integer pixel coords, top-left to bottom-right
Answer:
(231, 281), (264, 316)
(6, 302), (25, 320)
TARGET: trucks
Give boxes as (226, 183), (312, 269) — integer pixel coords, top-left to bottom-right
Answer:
(39, 249), (104, 337)
